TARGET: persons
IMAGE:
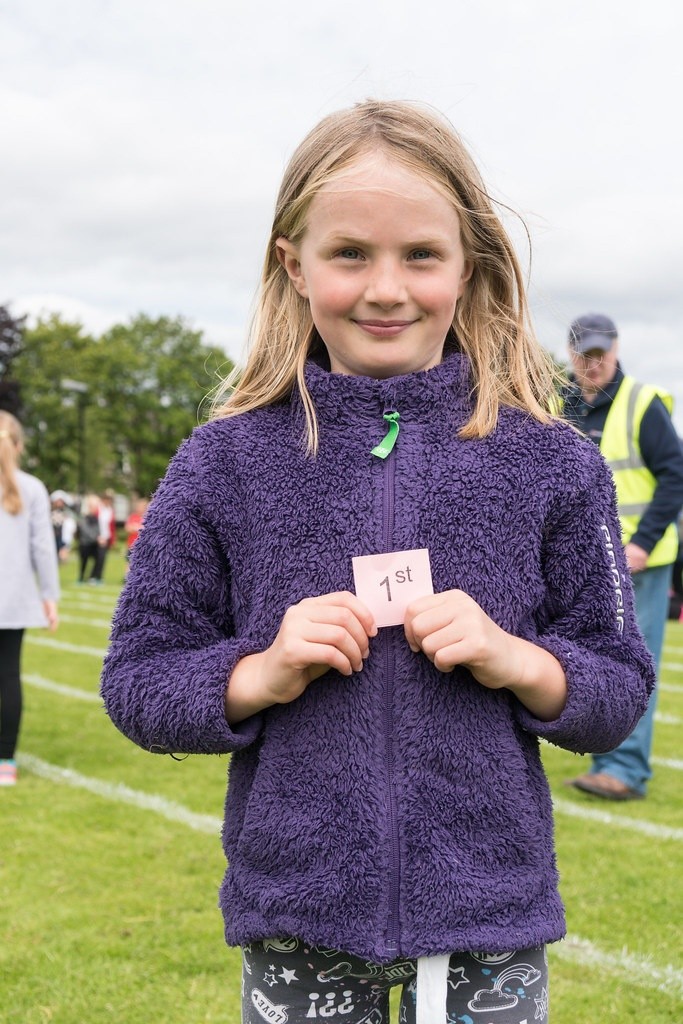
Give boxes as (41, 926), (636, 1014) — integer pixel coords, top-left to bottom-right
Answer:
(541, 314), (683, 802)
(101, 103), (656, 1024)
(0, 407), (59, 784)
(49, 489), (150, 585)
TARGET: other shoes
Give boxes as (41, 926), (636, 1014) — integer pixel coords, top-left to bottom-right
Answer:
(0, 759), (18, 786)
(89, 578), (102, 585)
(573, 771), (636, 798)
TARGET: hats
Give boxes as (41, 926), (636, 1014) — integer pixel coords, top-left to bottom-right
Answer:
(104, 488), (114, 499)
(570, 315), (616, 352)
